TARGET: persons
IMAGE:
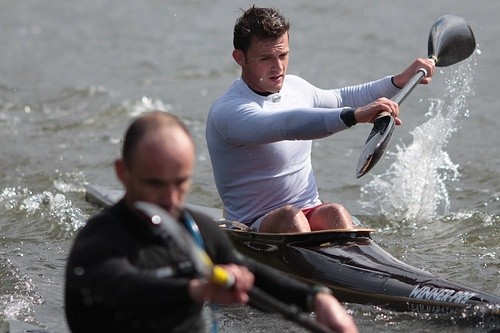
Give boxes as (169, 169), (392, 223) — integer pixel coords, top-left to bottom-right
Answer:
(62, 109), (359, 333)
(203, 4), (436, 238)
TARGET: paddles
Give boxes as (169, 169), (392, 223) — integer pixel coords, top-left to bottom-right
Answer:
(356, 13), (477, 177)
(136, 200), (333, 333)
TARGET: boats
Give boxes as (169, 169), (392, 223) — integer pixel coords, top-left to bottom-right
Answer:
(84, 185), (500, 325)
